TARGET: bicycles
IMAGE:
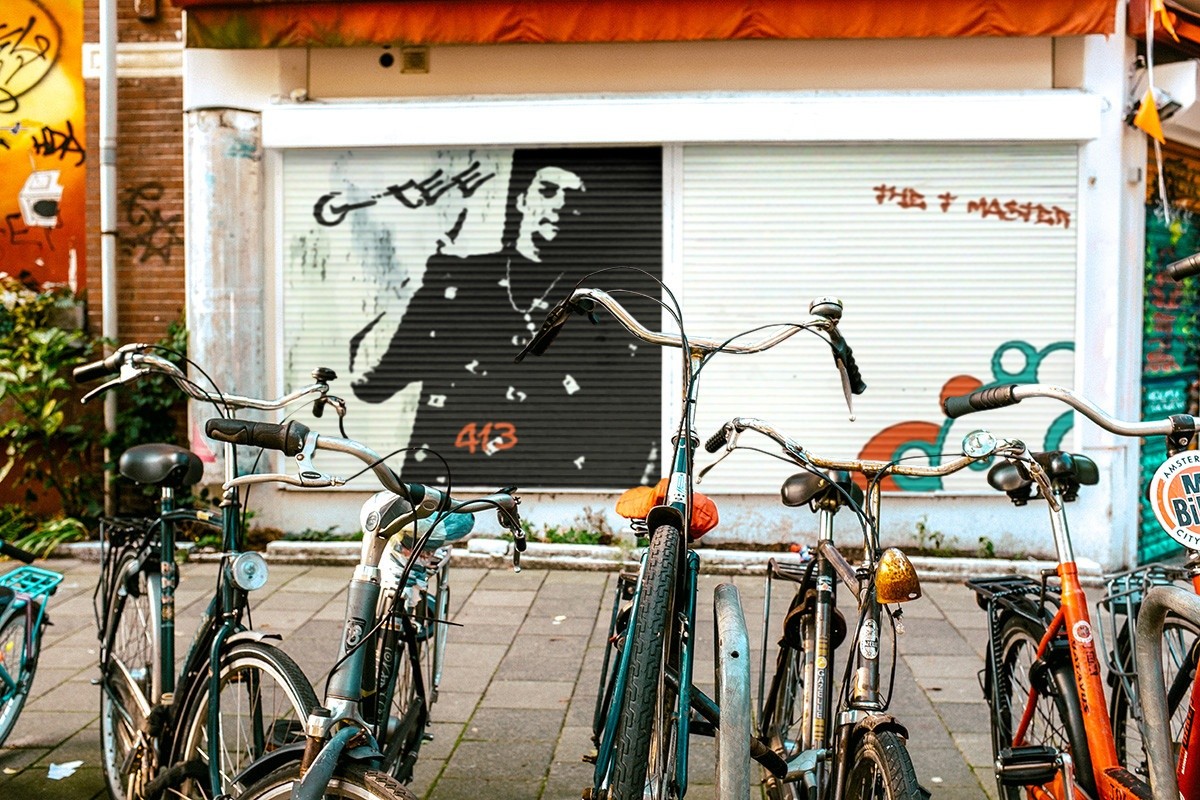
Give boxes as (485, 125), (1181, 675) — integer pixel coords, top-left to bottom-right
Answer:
(513, 264), (867, 800)
(941, 381), (1200, 800)
(698, 418), (1064, 799)
(67, 338), (349, 800)
(0, 423), (65, 756)
(198, 411), (518, 800)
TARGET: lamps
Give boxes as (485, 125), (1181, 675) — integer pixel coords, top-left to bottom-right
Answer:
(1141, 85), (1181, 121)
